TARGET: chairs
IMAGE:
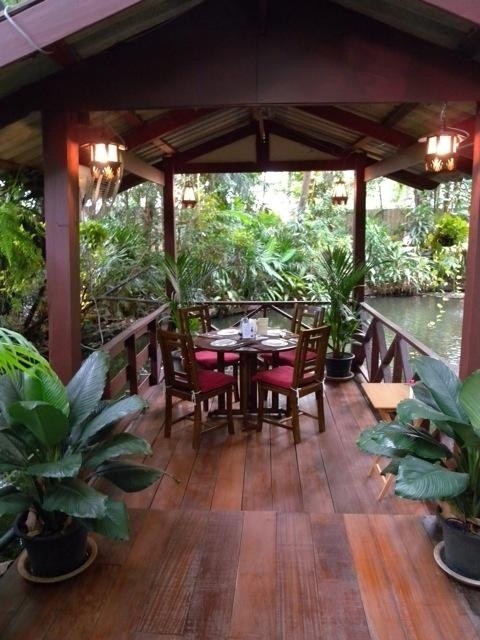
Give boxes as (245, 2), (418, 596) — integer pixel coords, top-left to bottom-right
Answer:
(178, 304), (241, 413)
(262, 302), (326, 410)
(252, 325), (333, 444)
(157, 328), (236, 450)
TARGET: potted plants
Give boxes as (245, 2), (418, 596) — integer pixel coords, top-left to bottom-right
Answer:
(355, 355), (480, 582)
(0, 349), (179, 583)
(312, 247), (392, 378)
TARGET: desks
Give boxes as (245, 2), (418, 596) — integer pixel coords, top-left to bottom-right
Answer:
(358, 381), (428, 502)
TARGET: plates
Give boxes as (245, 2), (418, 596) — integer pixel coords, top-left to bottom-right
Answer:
(261, 338), (290, 349)
(216, 328), (240, 337)
(210, 338), (239, 347)
(265, 329), (287, 337)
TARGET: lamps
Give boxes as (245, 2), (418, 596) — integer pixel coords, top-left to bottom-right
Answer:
(418, 101), (470, 173)
(330, 176), (348, 205)
(82, 120), (127, 180)
(181, 181), (198, 210)
